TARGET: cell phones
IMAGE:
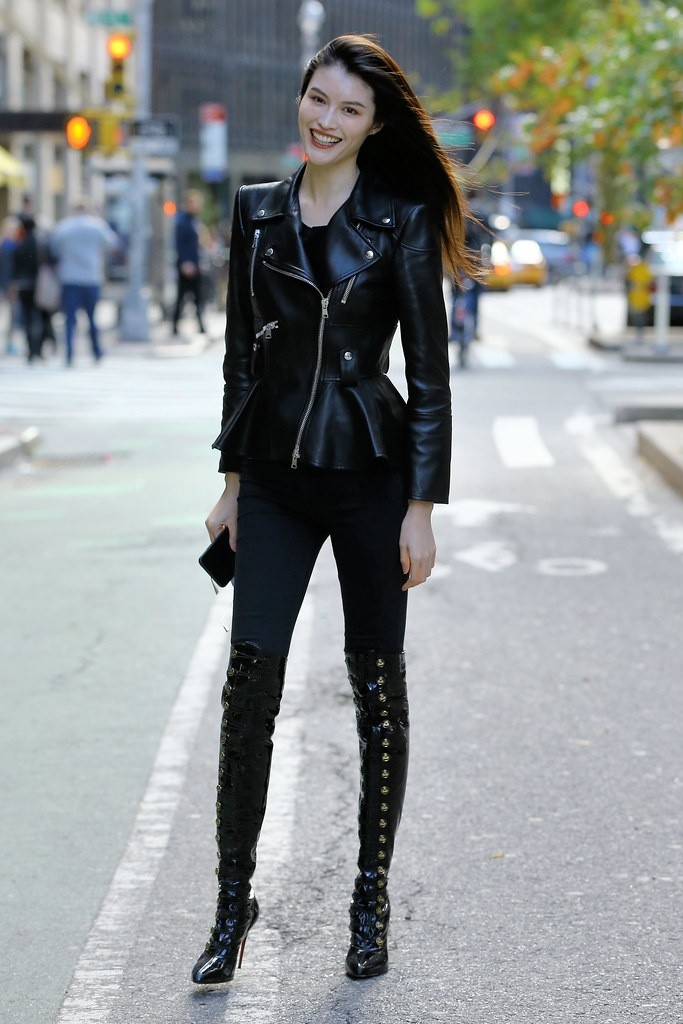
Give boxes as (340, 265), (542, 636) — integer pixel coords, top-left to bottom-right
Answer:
(198, 526), (236, 588)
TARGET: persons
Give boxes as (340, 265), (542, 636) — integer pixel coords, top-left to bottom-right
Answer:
(448, 185), (496, 369)
(181, 34), (491, 983)
(169, 189), (207, 337)
(0, 203), (124, 368)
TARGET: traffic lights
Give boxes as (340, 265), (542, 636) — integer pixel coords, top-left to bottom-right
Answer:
(105, 29), (132, 101)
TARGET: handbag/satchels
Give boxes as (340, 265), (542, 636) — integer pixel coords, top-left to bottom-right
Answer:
(33, 264), (62, 312)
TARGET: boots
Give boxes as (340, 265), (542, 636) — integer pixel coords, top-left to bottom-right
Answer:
(343, 646), (409, 980)
(192, 644), (287, 984)
